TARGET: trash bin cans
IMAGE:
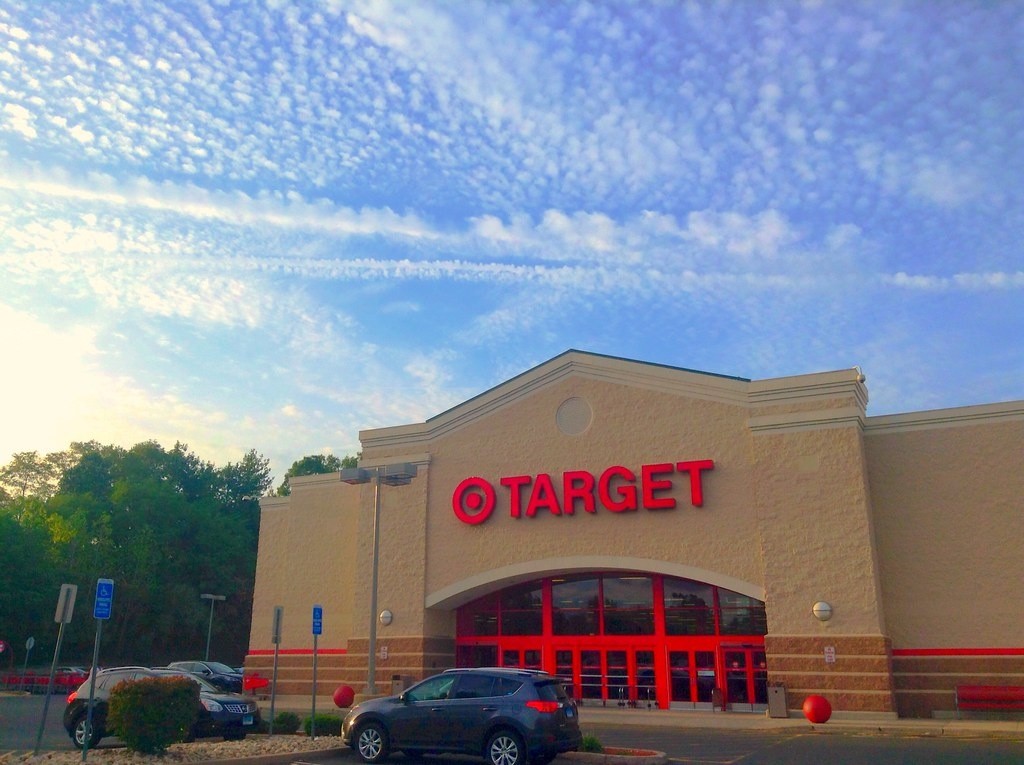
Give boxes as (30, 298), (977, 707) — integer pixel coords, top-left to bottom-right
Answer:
(766, 680), (790, 718)
(391, 674), (415, 696)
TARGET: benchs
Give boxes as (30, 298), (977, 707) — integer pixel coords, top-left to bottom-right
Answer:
(954, 686), (1024, 720)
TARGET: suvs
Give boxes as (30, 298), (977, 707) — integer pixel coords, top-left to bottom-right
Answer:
(167, 661), (243, 694)
(341, 667), (584, 765)
(63, 666), (259, 750)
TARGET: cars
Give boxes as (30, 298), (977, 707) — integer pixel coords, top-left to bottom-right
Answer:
(33, 666), (91, 695)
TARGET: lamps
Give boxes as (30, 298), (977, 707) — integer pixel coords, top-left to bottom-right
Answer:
(380, 610), (392, 626)
(812, 601), (832, 621)
